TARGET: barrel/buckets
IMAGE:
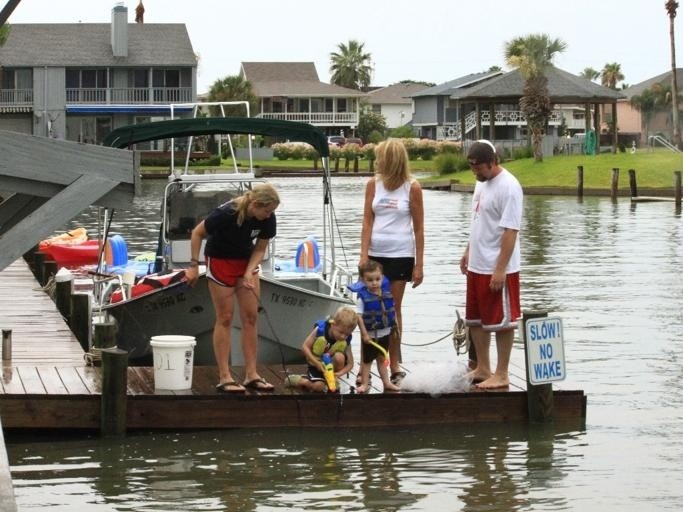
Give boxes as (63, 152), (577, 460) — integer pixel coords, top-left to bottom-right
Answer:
(149, 334), (197, 390)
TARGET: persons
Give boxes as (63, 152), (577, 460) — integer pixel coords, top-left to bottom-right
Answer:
(184, 183), (280, 393)
(460, 139), (523, 388)
(284, 305), (357, 392)
(356, 138), (423, 384)
(355, 260), (400, 392)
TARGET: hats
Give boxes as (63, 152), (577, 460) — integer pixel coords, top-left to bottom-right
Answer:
(467, 139), (496, 165)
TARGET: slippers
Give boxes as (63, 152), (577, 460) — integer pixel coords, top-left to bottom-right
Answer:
(391, 372), (406, 385)
(216, 381), (246, 392)
(356, 373), (372, 386)
(243, 378), (275, 390)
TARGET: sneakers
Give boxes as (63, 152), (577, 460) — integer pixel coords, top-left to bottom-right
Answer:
(284, 375), (301, 387)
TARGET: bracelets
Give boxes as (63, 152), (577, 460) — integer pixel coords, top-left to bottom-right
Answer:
(190, 258), (199, 267)
